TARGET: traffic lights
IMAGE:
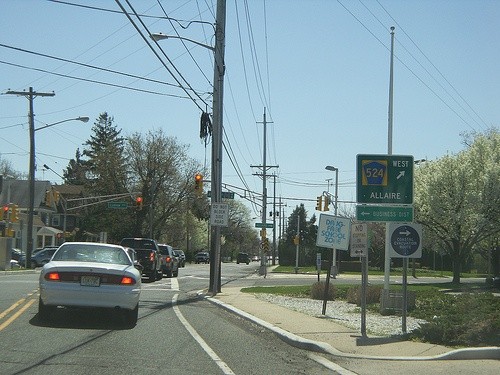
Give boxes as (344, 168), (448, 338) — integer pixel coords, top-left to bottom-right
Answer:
(44, 190), (51, 202)
(194, 174), (202, 192)
(136, 196), (143, 212)
(315, 196), (322, 211)
(54, 189), (59, 202)
(2, 205), (9, 220)
(12, 205), (20, 221)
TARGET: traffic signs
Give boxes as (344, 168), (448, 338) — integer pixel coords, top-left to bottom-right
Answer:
(356, 205), (415, 223)
(356, 153), (414, 205)
(389, 223), (422, 258)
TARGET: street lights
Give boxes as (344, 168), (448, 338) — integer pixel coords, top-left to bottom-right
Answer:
(324, 166), (339, 279)
(148, 31), (225, 295)
(25, 116), (90, 269)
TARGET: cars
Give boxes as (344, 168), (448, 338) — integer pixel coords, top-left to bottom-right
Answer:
(173, 249), (186, 268)
(38, 242), (142, 325)
(10, 246), (59, 269)
(252, 256), (279, 262)
(123, 247), (144, 276)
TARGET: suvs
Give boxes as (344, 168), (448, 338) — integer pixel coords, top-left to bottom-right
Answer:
(237, 253), (249, 265)
(143, 244), (179, 278)
(195, 251), (209, 264)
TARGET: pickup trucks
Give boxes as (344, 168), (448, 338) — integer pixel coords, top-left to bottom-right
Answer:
(118, 238), (162, 282)
